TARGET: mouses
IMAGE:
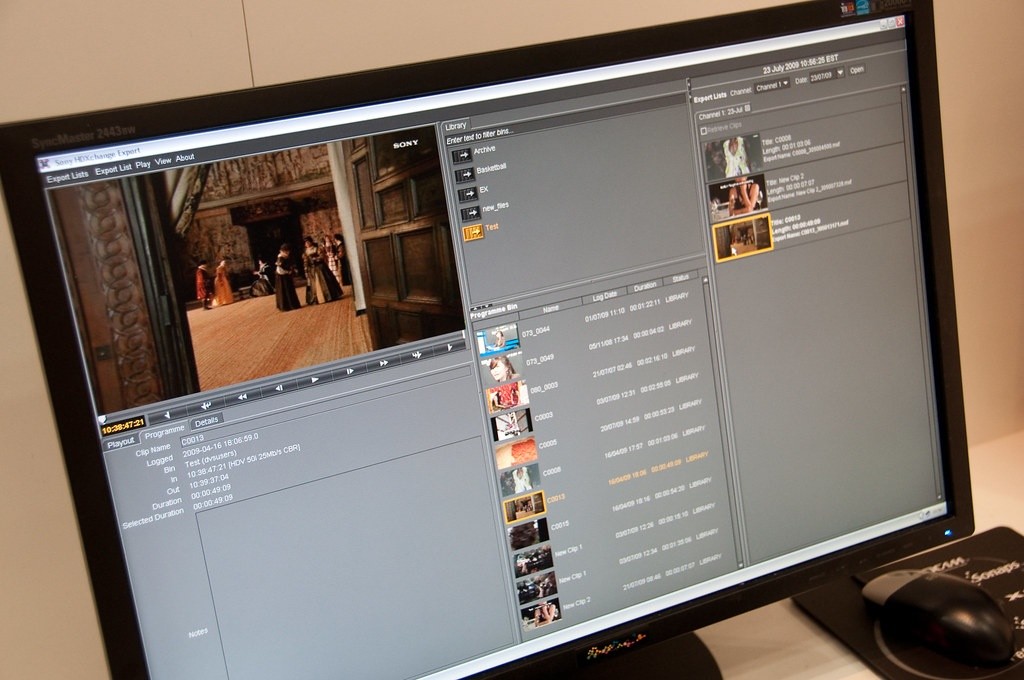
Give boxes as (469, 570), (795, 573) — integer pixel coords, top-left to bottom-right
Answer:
(878, 572), (1018, 677)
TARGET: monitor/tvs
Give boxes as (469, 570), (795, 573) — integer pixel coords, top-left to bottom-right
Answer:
(0, 0), (976, 680)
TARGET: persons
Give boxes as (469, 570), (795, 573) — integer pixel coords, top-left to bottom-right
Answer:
(505, 414), (517, 430)
(500, 466), (539, 496)
(492, 389), (521, 412)
(707, 135), (761, 177)
(516, 573), (558, 605)
(509, 521), (537, 550)
(523, 505), (533, 512)
(493, 331), (506, 349)
(197, 234), (351, 311)
(490, 356), (520, 383)
(731, 229), (754, 248)
(517, 549), (552, 577)
(535, 601), (556, 627)
(711, 176), (763, 219)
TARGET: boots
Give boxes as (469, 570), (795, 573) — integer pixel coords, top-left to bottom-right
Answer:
(203, 300), (212, 310)
